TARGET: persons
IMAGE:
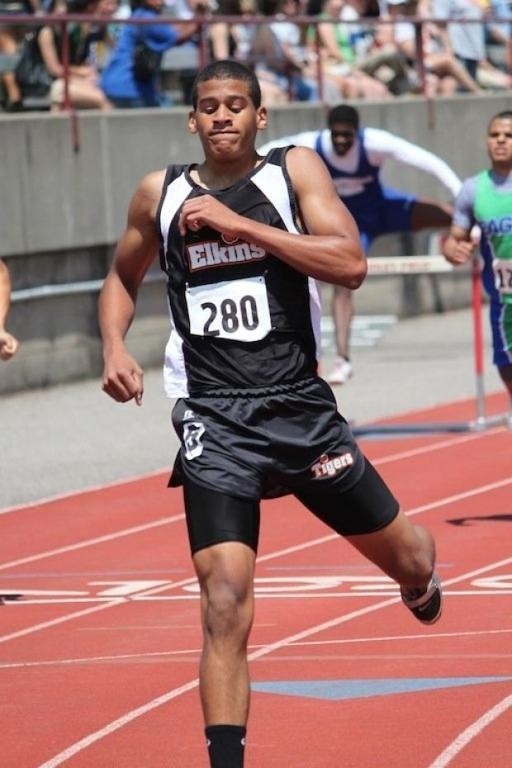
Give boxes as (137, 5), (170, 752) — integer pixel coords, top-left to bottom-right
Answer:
(257, 105), (465, 384)
(99, 61), (443, 768)
(1, 0), (512, 112)
(444, 111), (512, 402)
(0, 258), (18, 360)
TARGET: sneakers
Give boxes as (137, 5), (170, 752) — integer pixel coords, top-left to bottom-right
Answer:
(327, 358), (354, 385)
(400, 573), (443, 626)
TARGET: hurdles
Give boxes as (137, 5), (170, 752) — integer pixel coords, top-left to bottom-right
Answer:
(350, 246), (508, 435)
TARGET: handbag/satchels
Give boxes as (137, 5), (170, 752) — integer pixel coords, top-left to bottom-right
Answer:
(15, 38), (107, 107)
(134, 46), (162, 79)
